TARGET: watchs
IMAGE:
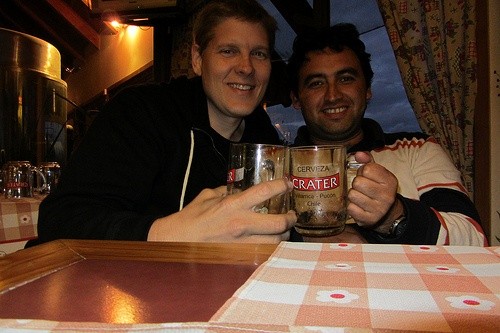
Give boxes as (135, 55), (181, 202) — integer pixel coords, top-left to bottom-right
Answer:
(373, 208), (406, 238)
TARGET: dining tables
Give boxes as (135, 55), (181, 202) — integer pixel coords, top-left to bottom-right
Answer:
(0, 239), (500, 333)
(0, 195), (47, 244)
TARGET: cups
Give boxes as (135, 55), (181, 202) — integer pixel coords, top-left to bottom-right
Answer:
(36, 162), (61, 194)
(290, 144), (367, 238)
(226, 142), (291, 241)
(2, 160), (47, 201)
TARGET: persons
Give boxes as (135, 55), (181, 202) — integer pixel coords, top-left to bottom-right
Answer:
(290, 22), (488, 246)
(37, 0), (298, 243)
